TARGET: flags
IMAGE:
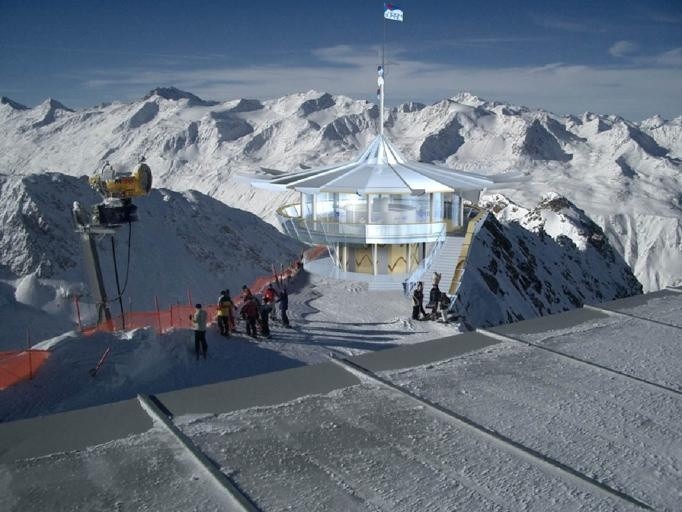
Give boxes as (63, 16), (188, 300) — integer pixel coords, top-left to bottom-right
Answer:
(384, 2), (405, 22)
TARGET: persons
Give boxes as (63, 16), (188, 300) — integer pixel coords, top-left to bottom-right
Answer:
(215, 284), (289, 338)
(401, 270), (452, 324)
(188, 303), (208, 362)
